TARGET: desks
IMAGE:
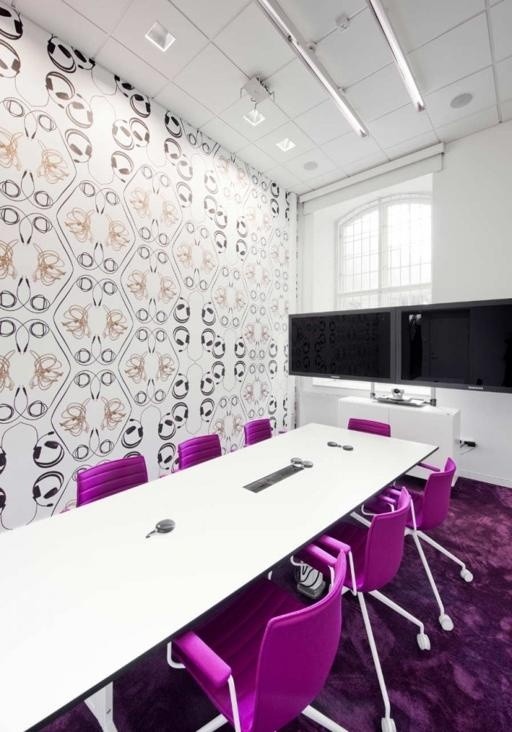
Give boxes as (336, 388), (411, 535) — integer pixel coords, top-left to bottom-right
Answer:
(0, 421), (440, 731)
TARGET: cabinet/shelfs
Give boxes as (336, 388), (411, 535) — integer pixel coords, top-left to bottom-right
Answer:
(337, 394), (461, 495)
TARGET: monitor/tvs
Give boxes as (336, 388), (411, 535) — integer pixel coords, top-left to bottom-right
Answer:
(396, 297), (512, 393)
(288, 298), (395, 384)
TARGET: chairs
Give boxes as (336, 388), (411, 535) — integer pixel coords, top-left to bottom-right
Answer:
(360, 455), (475, 632)
(34, 700), (105, 731)
(75, 454), (149, 509)
(241, 419), (273, 447)
(165, 544), (350, 732)
(290, 487), (432, 732)
(177, 434), (222, 471)
(348, 418), (392, 436)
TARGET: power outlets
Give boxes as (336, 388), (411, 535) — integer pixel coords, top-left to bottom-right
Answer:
(460, 440), (476, 449)
